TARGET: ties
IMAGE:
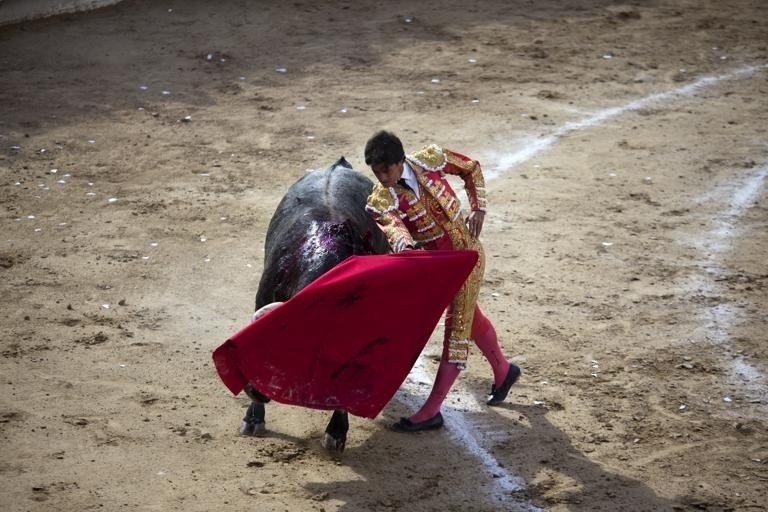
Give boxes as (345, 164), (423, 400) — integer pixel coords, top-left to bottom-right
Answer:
(397, 178), (416, 195)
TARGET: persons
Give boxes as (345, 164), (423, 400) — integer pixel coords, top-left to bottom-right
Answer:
(364, 131), (521, 431)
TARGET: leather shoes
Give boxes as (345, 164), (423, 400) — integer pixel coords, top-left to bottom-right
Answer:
(486, 364), (520, 405)
(392, 411), (443, 432)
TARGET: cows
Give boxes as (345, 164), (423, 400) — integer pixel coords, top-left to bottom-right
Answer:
(238, 155), (390, 453)
